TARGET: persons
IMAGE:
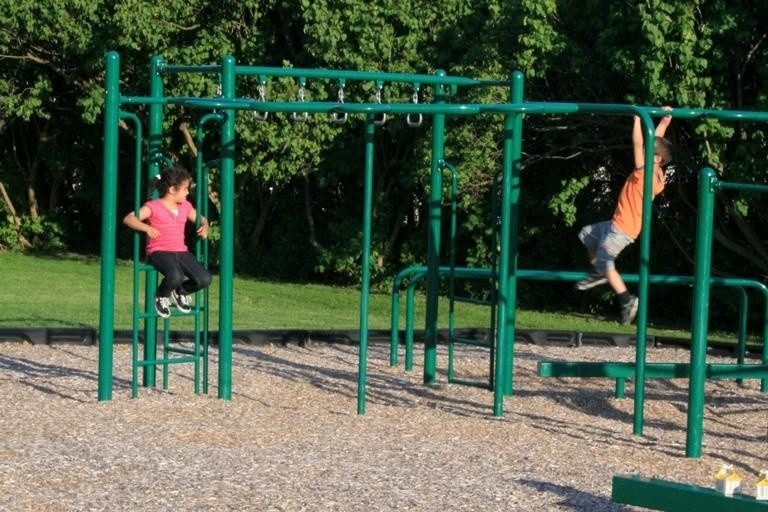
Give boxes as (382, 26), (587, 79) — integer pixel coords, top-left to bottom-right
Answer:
(573, 104), (677, 326)
(123, 165), (213, 318)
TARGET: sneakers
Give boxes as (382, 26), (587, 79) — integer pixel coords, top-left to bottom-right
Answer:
(575, 268), (609, 291)
(155, 294), (172, 319)
(170, 288), (192, 313)
(619, 294), (639, 329)
